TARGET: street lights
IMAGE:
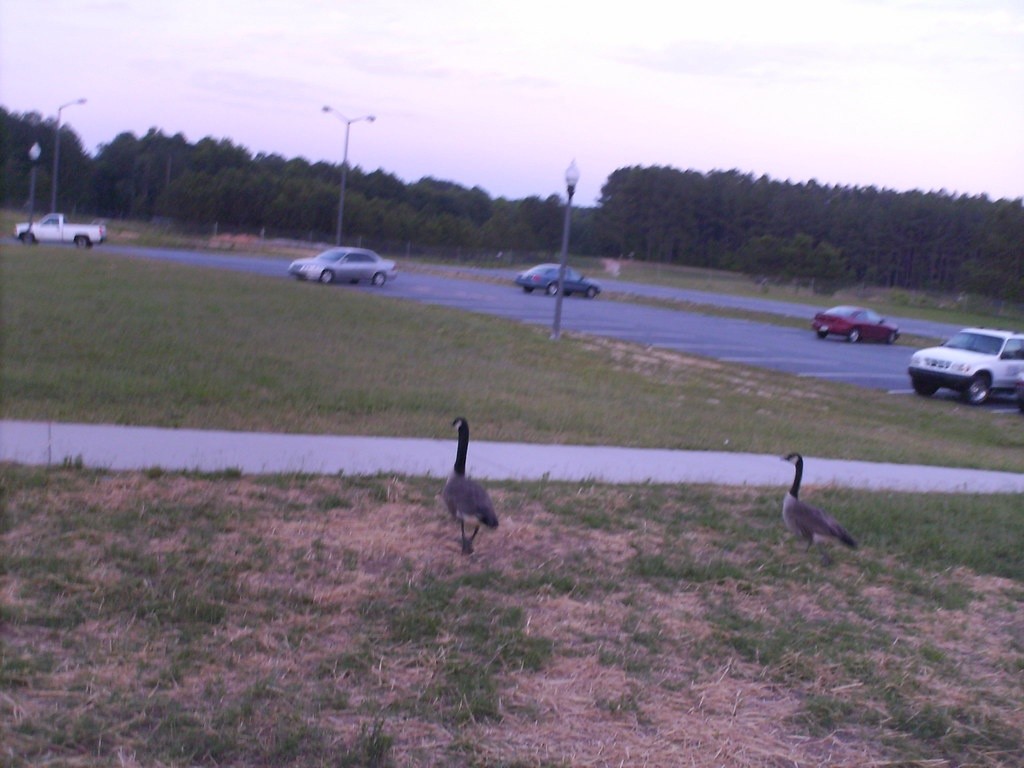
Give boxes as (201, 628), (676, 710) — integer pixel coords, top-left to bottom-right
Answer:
(550, 160), (580, 340)
(321, 105), (376, 247)
(23, 142), (41, 246)
(50, 98), (88, 212)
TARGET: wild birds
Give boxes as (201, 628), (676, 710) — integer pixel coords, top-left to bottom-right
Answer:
(780, 450), (858, 564)
(440, 415), (499, 558)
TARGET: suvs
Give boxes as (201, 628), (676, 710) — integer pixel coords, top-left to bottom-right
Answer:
(908, 326), (1024, 406)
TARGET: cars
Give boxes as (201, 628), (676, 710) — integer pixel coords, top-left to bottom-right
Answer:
(287, 246), (399, 289)
(514, 263), (602, 299)
(811, 305), (902, 345)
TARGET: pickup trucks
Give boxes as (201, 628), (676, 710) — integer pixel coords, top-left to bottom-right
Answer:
(11, 212), (107, 250)
